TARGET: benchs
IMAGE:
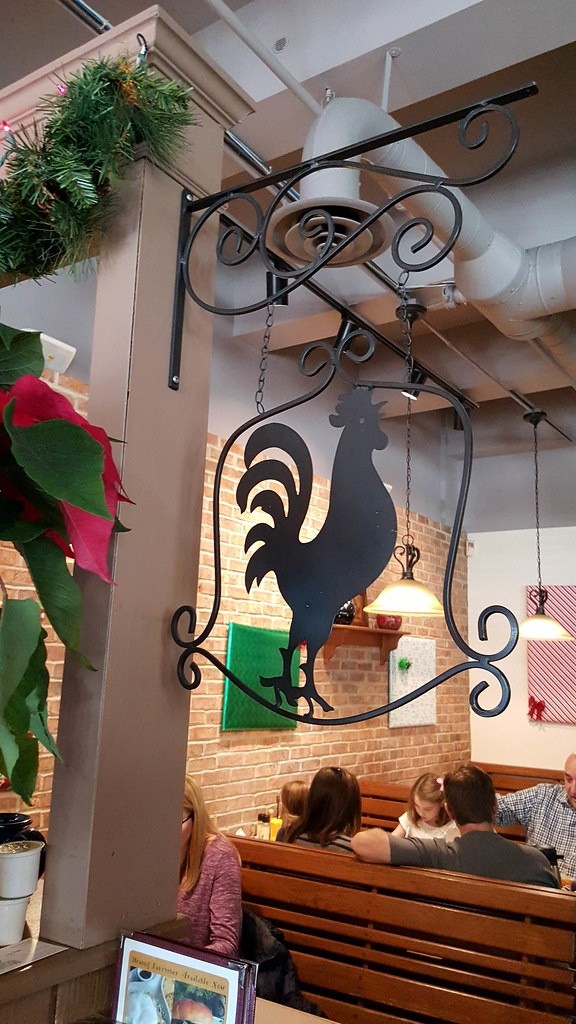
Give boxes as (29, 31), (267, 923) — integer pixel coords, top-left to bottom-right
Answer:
(223, 835), (576, 1024)
(357, 779), (528, 846)
(469, 761), (566, 796)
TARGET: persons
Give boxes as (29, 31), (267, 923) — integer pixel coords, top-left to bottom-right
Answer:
(275, 766), (362, 858)
(390, 773), (461, 843)
(280, 780), (310, 829)
(495, 751), (576, 880)
(177, 774), (242, 959)
(350, 762), (561, 889)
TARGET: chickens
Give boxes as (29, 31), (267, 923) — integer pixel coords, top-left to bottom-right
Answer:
(234, 388), (398, 720)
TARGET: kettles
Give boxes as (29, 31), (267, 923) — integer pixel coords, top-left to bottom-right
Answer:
(539, 845), (564, 889)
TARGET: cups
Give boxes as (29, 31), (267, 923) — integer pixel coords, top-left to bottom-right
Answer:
(271, 818), (282, 841)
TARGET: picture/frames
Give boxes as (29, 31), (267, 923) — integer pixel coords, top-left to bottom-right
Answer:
(110, 928), (259, 1024)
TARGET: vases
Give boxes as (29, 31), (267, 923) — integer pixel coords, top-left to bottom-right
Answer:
(0, 894), (33, 947)
(0, 840), (45, 899)
(0, 813), (47, 881)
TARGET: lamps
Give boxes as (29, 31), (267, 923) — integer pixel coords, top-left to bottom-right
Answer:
(509, 413), (574, 643)
(401, 373), (429, 401)
(266, 269), (288, 308)
(453, 406), (470, 430)
(362, 303), (444, 618)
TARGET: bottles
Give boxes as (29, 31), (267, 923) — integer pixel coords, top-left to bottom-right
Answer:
(260, 815), (270, 840)
(257, 813), (266, 838)
